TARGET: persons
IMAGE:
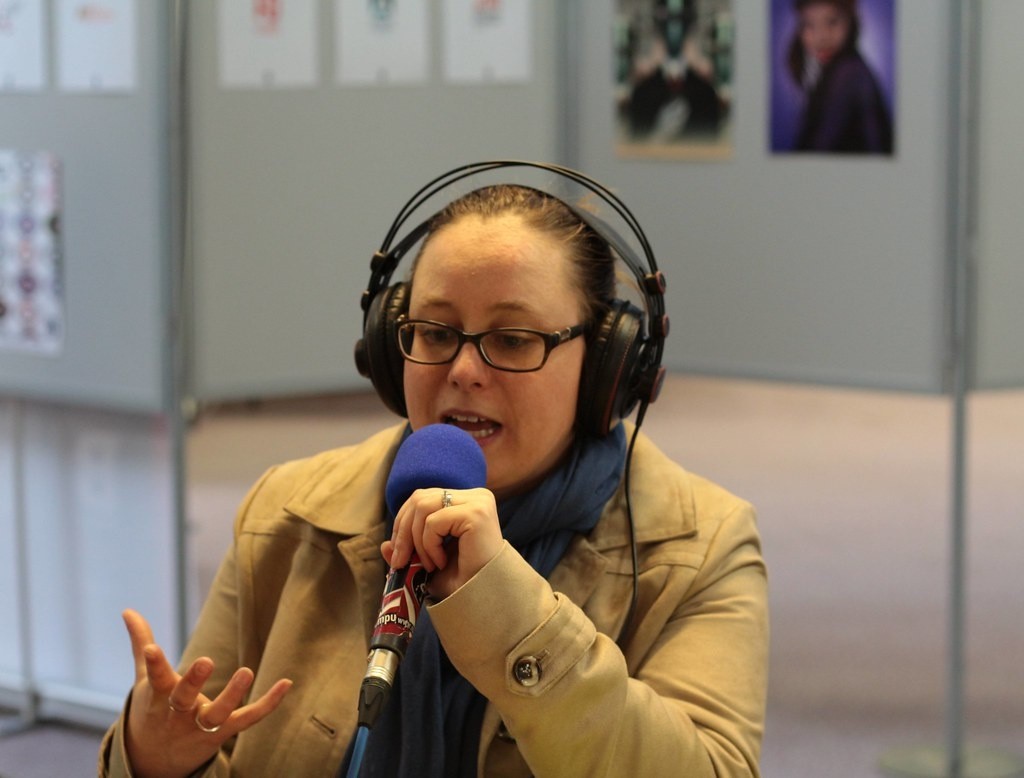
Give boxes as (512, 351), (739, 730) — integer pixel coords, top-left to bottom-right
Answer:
(621, 0), (724, 145)
(98, 184), (772, 778)
(785, 0), (890, 153)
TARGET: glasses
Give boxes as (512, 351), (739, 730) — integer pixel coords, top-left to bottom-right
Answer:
(393, 312), (594, 373)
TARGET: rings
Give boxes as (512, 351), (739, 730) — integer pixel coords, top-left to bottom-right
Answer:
(443, 491), (451, 508)
(168, 695), (197, 713)
(194, 704), (221, 732)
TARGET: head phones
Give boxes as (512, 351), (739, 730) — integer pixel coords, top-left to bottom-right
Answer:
(355, 160), (669, 438)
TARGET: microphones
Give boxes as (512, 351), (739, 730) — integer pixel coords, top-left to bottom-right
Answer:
(355, 423), (488, 732)
